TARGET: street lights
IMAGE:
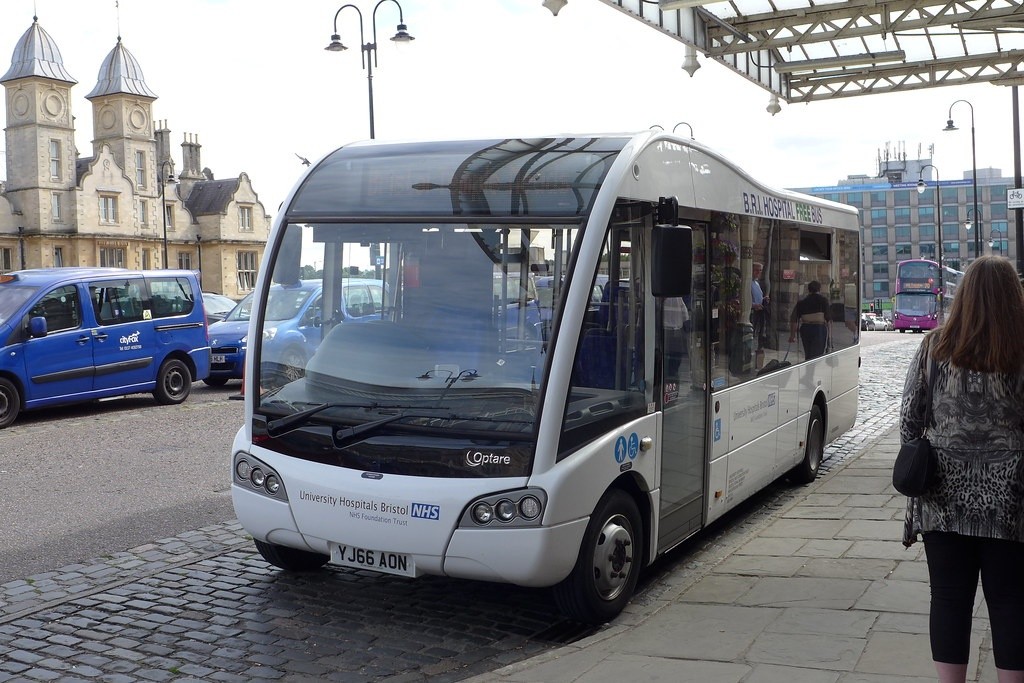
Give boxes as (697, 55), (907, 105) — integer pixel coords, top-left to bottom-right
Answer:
(942, 99), (980, 261)
(964, 208), (983, 256)
(162, 161), (178, 269)
(323, 0), (416, 140)
(987, 228), (1003, 256)
(917, 164), (945, 327)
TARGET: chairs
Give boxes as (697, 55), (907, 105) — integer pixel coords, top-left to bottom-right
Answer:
(44, 298), (70, 332)
(500, 280), (635, 388)
(99, 298), (177, 323)
(349, 293), (362, 316)
(416, 275), (481, 332)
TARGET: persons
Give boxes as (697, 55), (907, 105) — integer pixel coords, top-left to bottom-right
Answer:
(789, 280), (833, 360)
(892, 254), (1024, 683)
(750, 264), (770, 351)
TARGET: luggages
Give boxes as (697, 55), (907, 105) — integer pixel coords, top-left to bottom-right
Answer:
(756, 341), (795, 380)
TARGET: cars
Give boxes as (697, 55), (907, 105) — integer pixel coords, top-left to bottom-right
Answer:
(861, 314), (894, 331)
(201, 291), (240, 326)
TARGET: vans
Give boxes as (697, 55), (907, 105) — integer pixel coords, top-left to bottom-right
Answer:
(0, 267), (212, 431)
(599, 278), (689, 345)
(202, 279), (390, 389)
(493, 271), (543, 375)
(537, 275), (616, 346)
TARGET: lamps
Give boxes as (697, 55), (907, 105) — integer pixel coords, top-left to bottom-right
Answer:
(773, 50), (905, 74)
(658, 0), (735, 12)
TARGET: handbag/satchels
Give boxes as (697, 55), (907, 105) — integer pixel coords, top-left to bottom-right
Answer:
(892, 436), (934, 496)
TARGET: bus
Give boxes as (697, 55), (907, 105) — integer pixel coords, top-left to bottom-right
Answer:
(231, 131), (863, 622)
(892, 259), (966, 333)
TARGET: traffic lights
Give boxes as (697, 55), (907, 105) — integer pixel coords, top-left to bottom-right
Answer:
(870, 303), (874, 312)
